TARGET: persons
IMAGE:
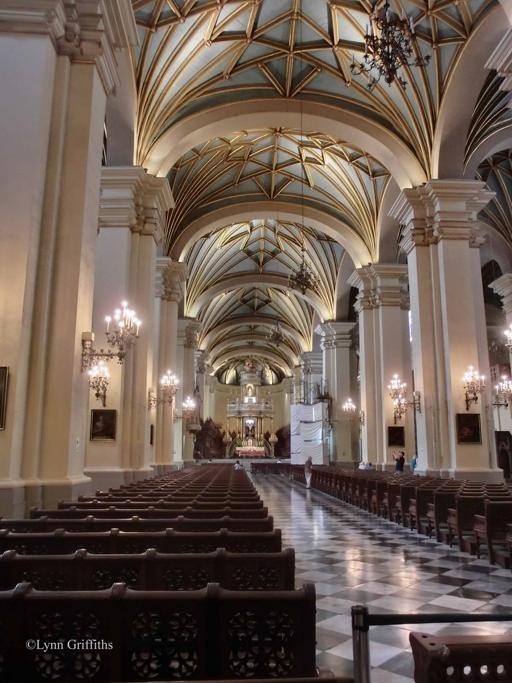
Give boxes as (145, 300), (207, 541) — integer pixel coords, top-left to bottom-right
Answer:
(364, 461), (374, 470)
(393, 450), (404, 473)
(394, 447), (401, 457)
(233, 460), (244, 470)
(207, 457), (213, 463)
(304, 455), (313, 488)
(93, 413), (115, 439)
(408, 452), (417, 472)
(277, 459), (282, 463)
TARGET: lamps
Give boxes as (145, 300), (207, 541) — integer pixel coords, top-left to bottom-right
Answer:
(84, 301), (154, 370)
(342, 397), (365, 425)
(87, 360), (114, 406)
(490, 370), (510, 412)
(282, 0), (322, 296)
(146, 367), (181, 411)
(170, 394), (197, 424)
(348, 0), (432, 97)
(459, 367), (484, 408)
(387, 370), (423, 422)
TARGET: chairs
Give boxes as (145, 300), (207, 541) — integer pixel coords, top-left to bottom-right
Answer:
(402, 630), (512, 682)
(315, 460), (512, 591)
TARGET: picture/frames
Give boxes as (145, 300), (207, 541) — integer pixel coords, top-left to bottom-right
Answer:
(456, 412), (482, 445)
(90, 408), (116, 440)
(388, 425), (404, 447)
(0, 364), (13, 431)
